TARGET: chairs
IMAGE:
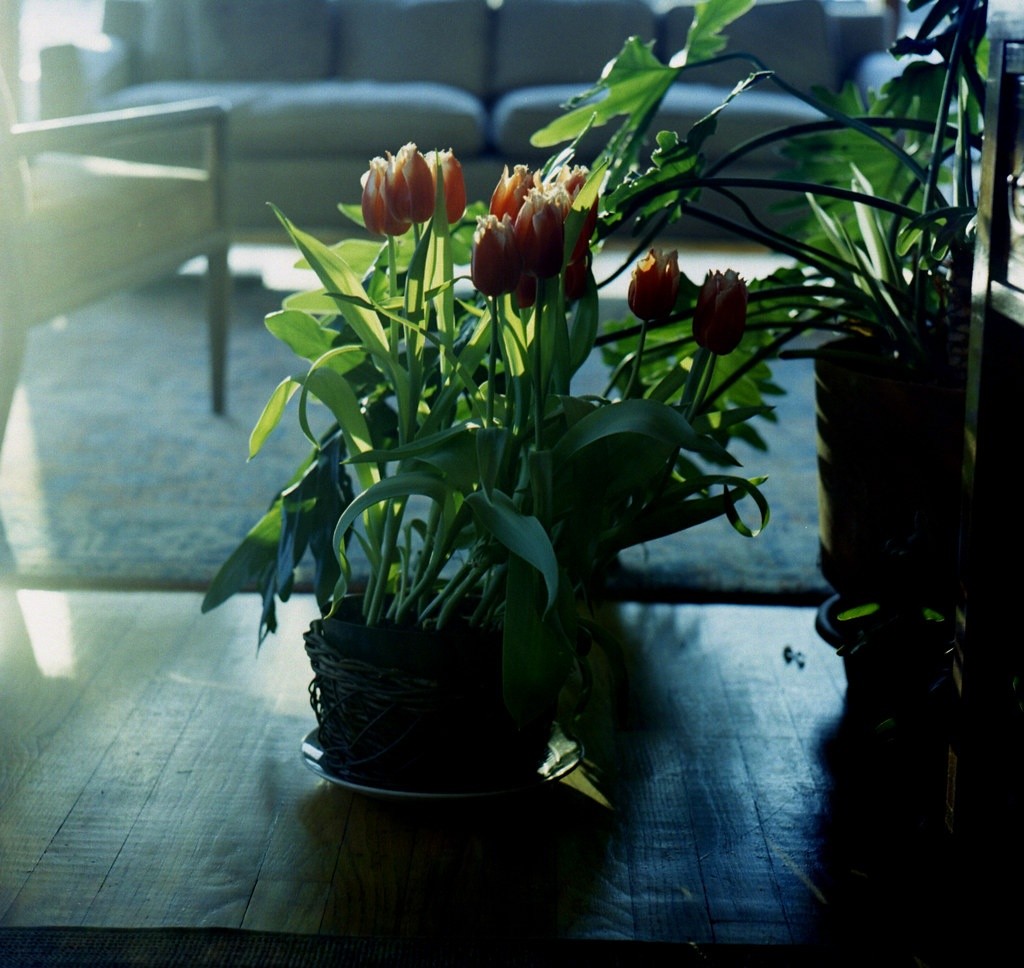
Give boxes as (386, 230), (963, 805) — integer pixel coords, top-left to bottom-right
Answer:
(0, 70), (233, 451)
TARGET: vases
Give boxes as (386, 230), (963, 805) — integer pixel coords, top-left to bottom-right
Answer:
(301, 598), (541, 787)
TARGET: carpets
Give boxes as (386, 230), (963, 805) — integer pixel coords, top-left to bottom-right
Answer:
(0, 288), (849, 601)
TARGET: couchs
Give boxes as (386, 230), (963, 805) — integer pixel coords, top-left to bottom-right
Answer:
(39, 0), (893, 244)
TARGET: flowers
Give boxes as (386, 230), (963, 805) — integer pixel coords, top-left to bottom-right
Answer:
(198, 139), (771, 770)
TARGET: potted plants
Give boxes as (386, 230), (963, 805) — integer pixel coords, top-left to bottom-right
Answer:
(530, 0), (991, 595)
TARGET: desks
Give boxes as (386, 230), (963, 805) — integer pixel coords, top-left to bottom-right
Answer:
(944, 37), (1024, 834)
